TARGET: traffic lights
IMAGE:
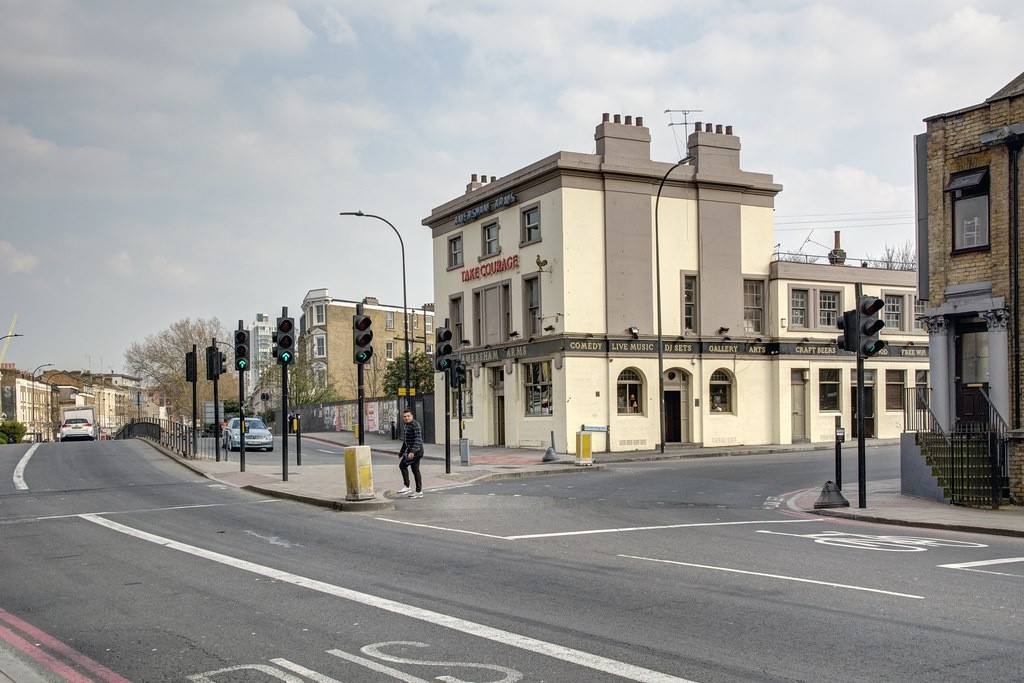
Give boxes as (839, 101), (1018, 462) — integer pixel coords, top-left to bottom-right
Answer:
(217, 352), (226, 374)
(835, 310), (857, 353)
(352, 315), (374, 364)
(857, 295), (887, 358)
(271, 331), (279, 358)
(276, 318), (295, 365)
(234, 330), (250, 370)
(456, 362), (466, 384)
(434, 327), (453, 371)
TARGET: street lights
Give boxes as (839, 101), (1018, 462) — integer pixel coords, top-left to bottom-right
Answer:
(654, 154), (697, 453)
(46, 372), (64, 442)
(339, 211), (412, 413)
(32, 363), (55, 435)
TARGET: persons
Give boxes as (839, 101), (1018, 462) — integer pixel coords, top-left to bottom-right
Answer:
(397, 409), (424, 499)
(287, 410), (296, 433)
(221, 418), (228, 448)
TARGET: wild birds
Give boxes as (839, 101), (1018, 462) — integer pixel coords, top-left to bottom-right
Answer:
(536, 255), (547, 272)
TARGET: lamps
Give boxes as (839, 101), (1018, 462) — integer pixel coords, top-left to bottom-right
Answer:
(802, 338), (810, 342)
(884, 341), (888, 345)
(587, 334), (594, 337)
(755, 338), (762, 342)
(461, 340), (470, 344)
(909, 341), (914, 345)
(484, 344), (492, 349)
(629, 326), (639, 339)
(528, 338), (534, 342)
(719, 326), (729, 333)
(724, 337), (732, 341)
(803, 370), (809, 381)
(543, 326), (554, 332)
(678, 336), (685, 340)
(830, 338), (836, 343)
(509, 331), (518, 337)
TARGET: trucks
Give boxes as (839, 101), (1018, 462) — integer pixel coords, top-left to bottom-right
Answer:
(59, 405), (99, 441)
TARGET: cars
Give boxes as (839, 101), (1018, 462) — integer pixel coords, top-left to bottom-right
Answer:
(221, 417), (274, 451)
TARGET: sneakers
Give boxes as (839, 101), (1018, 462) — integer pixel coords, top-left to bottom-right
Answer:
(408, 491), (423, 499)
(397, 485), (412, 495)
